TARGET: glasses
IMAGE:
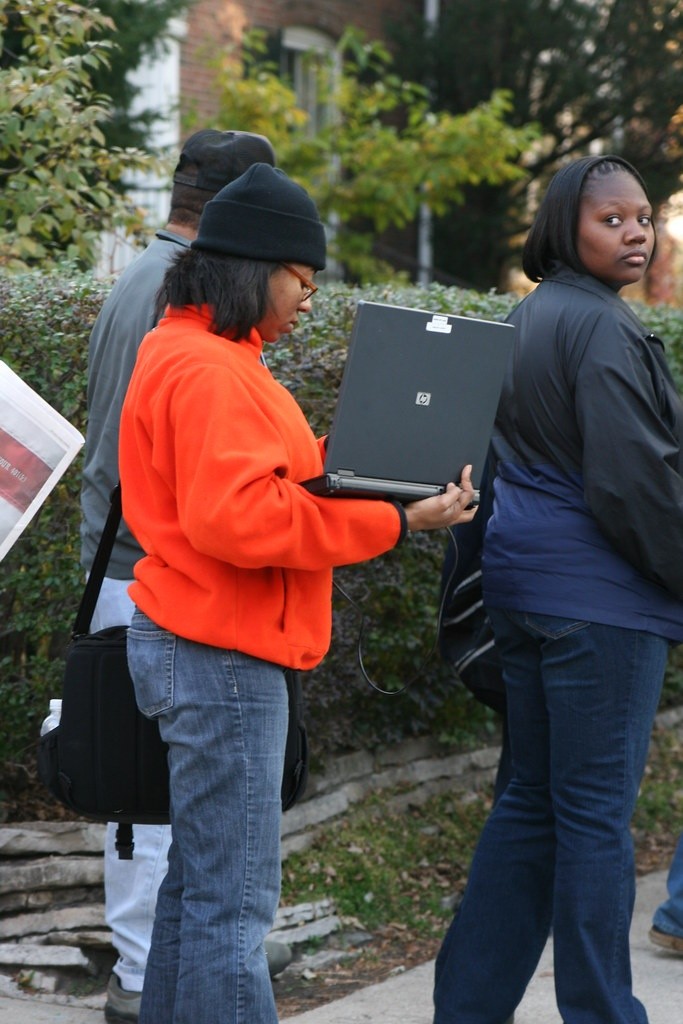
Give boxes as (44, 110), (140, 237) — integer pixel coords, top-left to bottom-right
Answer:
(279, 261), (319, 302)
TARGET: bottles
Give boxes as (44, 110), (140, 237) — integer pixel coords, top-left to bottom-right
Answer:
(41, 697), (62, 738)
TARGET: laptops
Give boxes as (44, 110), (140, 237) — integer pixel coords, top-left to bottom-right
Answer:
(301, 299), (513, 502)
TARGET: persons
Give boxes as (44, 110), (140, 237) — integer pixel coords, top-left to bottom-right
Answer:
(79, 129), (291, 1024)
(433, 154), (683, 1024)
(647, 830), (682, 951)
(119, 163), (479, 1024)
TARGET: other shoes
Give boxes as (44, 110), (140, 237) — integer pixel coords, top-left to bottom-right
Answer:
(264, 939), (292, 978)
(104, 973), (144, 1023)
(649, 925), (682, 953)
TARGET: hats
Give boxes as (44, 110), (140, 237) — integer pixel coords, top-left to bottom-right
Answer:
(191, 163), (326, 272)
(173, 129), (277, 192)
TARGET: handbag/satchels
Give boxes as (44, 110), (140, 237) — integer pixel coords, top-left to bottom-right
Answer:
(37, 625), (312, 825)
(437, 513), (507, 716)
